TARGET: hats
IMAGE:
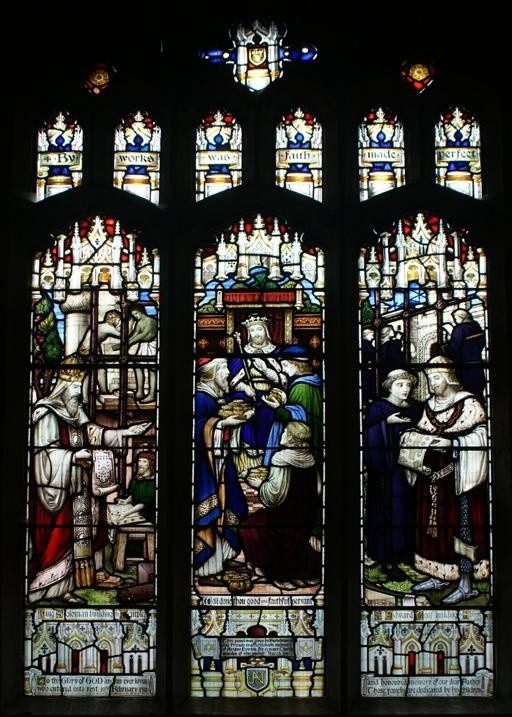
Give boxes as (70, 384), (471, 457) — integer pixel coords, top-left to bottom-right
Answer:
(274, 344), (311, 362)
(197, 356), (229, 372)
(421, 355), (464, 393)
(381, 369), (418, 388)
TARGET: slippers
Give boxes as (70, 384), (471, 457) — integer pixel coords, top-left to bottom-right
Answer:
(61, 591), (89, 606)
(408, 577), (480, 606)
